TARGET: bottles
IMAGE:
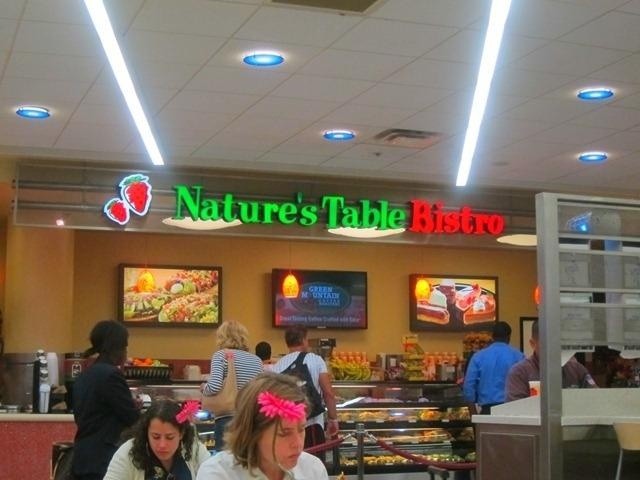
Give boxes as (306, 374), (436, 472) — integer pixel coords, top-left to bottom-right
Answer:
(434, 351), (442, 364)
(338, 351), (347, 361)
(347, 351), (354, 362)
(354, 351), (361, 364)
(461, 407), (470, 419)
(361, 351), (368, 362)
(443, 351), (450, 361)
(449, 351), (460, 365)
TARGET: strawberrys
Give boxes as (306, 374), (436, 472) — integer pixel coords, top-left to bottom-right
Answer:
(106, 199), (128, 225)
(119, 175), (153, 216)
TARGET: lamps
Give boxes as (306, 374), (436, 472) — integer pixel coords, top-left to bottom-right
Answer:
(577, 87), (614, 100)
(323, 129), (355, 140)
(15, 105), (50, 117)
(282, 239), (299, 298)
(579, 152), (608, 161)
(244, 52), (283, 66)
(415, 244), (430, 301)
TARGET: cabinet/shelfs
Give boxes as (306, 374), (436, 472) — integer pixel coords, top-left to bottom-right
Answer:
(326, 379), (477, 475)
(128, 381), (215, 457)
(472, 192), (640, 480)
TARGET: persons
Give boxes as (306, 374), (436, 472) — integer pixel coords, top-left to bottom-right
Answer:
(197, 319), (265, 452)
(504, 319), (592, 402)
(196, 372), (321, 480)
(101, 398), (212, 480)
(69, 319), (146, 480)
(269, 325), (339, 466)
(461, 321), (518, 439)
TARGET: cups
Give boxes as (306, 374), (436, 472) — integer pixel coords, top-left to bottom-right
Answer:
(529, 380), (540, 396)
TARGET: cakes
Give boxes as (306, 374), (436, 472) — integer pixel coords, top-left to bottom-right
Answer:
(359, 411), (389, 421)
(420, 410), (442, 421)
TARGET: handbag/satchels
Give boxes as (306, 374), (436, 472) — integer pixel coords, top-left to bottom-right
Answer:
(49, 438), (74, 480)
(201, 349), (237, 414)
